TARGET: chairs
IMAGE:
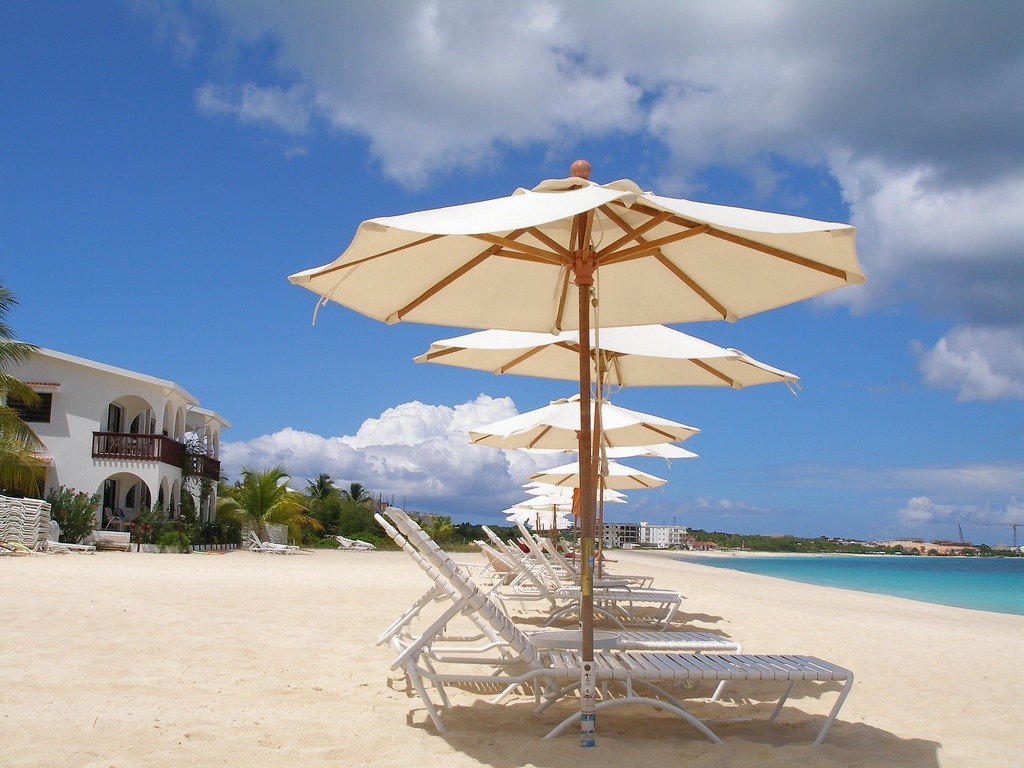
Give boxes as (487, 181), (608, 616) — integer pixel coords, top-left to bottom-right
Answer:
(375, 506), (854, 747)
(250, 531), (298, 556)
(335, 535), (376, 552)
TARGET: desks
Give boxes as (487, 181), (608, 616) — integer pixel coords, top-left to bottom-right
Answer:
(120, 446), (147, 455)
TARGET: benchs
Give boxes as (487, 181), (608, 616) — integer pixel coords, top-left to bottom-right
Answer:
(91, 529), (130, 552)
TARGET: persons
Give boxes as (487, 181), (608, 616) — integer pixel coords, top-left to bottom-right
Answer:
(594, 551), (618, 563)
(557, 545), (581, 559)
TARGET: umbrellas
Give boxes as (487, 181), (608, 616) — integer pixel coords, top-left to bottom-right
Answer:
(289, 159), (869, 749)
(502, 457), (668, 567)
(513, 443), (695, 579)
(469, 393), (701, 573)
(413, 325), (801, 563)
